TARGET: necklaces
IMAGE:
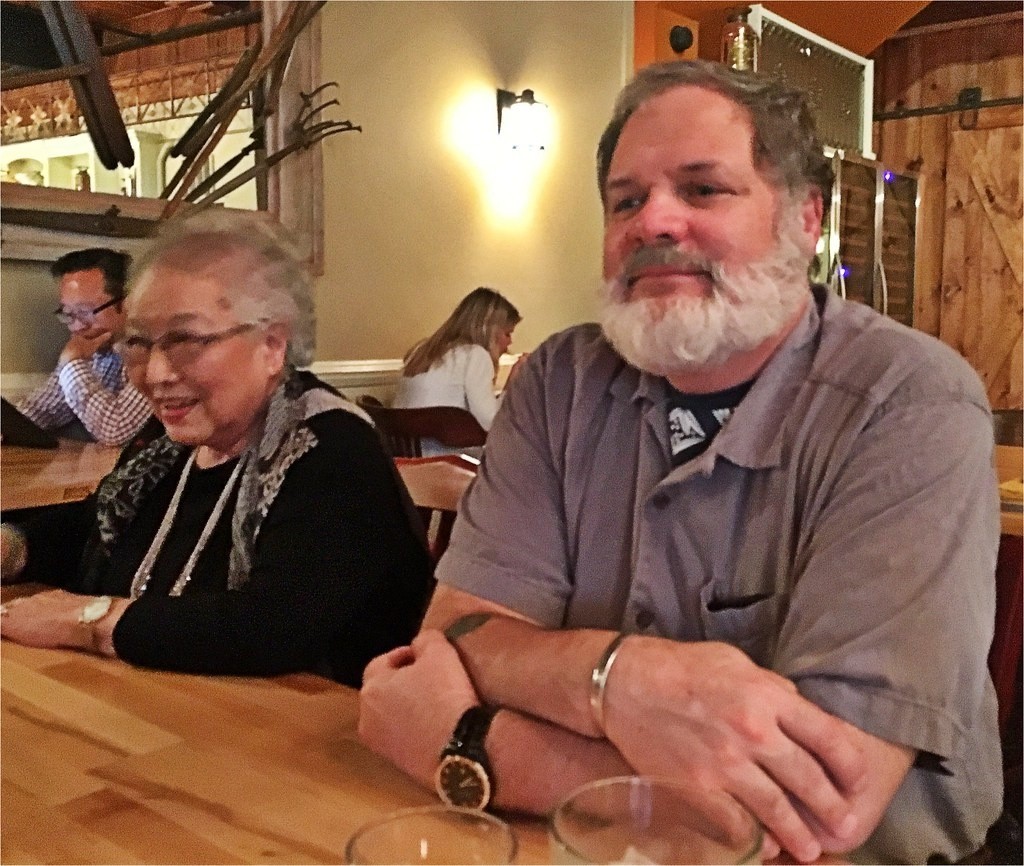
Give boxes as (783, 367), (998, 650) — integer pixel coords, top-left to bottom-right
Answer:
(196, 462), (209, 469)
(131, 444), (250, 596)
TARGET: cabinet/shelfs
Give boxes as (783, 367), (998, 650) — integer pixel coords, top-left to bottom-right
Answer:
(820, 147), (927, 332)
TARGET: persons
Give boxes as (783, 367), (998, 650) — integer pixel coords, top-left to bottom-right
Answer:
(393, 286), (522, 561)
(356, 61), (1005, 863)
(0, 208), (435, 690)
(0, 245), (167, 444)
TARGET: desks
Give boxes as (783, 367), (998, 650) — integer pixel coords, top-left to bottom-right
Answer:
(0, 435), (129, 510)
(994, 444), (1023, 748)
(1, 582), (850, 866)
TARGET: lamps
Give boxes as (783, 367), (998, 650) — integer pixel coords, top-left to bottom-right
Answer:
(719, 6), (761, 71)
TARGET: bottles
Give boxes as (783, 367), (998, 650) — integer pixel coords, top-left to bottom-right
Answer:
(76, 165), (91, 192)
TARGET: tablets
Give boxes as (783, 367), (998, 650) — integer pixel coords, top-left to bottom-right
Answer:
(0, 396), (60, 450)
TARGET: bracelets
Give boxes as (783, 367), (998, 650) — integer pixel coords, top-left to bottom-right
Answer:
(1, 521), (24, 577)
(589, 629), (636, 737)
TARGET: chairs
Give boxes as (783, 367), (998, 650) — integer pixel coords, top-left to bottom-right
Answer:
(390, 453), (482, 566)
(354, 395), (490, 456)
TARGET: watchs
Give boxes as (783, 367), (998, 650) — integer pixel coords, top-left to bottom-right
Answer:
(79, 595), (120, 655)
(435, 704), (503, 820)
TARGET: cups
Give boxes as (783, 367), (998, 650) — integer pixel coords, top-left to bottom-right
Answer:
(343, 804), (520, 866)
(549, 775), (767, 866)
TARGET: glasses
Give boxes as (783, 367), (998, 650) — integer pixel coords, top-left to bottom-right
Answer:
(113, 319), (268, 366)
(55, 294), (123, 325)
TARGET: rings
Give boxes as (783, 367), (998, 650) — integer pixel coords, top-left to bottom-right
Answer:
(0, 604), (8, 614)
